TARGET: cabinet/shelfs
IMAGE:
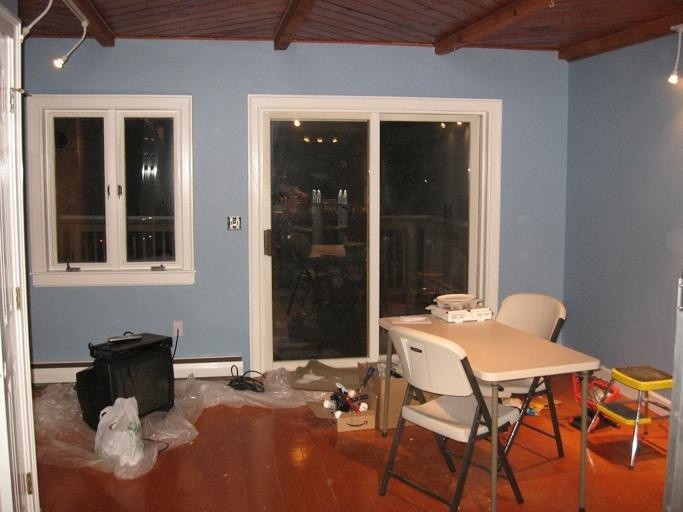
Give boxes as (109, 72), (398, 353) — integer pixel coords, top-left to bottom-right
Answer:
(126, 116), (161, 260)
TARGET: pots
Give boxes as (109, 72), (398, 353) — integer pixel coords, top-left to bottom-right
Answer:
(433, 293), (478, 310)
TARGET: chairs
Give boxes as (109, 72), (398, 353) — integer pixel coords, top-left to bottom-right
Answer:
(287, 230), (349, 326)
(458, 293), (569, 470)
(379, 323), (528, 511)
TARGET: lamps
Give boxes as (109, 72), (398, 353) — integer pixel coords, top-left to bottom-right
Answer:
(51, 20), (89, 68)
(667, 23), (683, 86)
(18, 0), (53, 44)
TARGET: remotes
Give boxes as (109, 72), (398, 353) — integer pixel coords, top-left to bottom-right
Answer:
(108, 335), (143, 343)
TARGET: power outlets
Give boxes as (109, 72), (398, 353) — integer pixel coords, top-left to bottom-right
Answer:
(225, 215), (241, 231)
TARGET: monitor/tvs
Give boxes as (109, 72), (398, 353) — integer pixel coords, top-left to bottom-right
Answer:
(76, 348), (174, 431)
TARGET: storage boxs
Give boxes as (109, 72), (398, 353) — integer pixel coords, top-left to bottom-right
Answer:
(306, 358), (441, 435)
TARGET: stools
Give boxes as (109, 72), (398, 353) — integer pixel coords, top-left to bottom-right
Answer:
(418, 267), (445, 311)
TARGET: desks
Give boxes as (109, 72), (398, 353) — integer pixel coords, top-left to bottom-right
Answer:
(378, 304), (598, 510)
